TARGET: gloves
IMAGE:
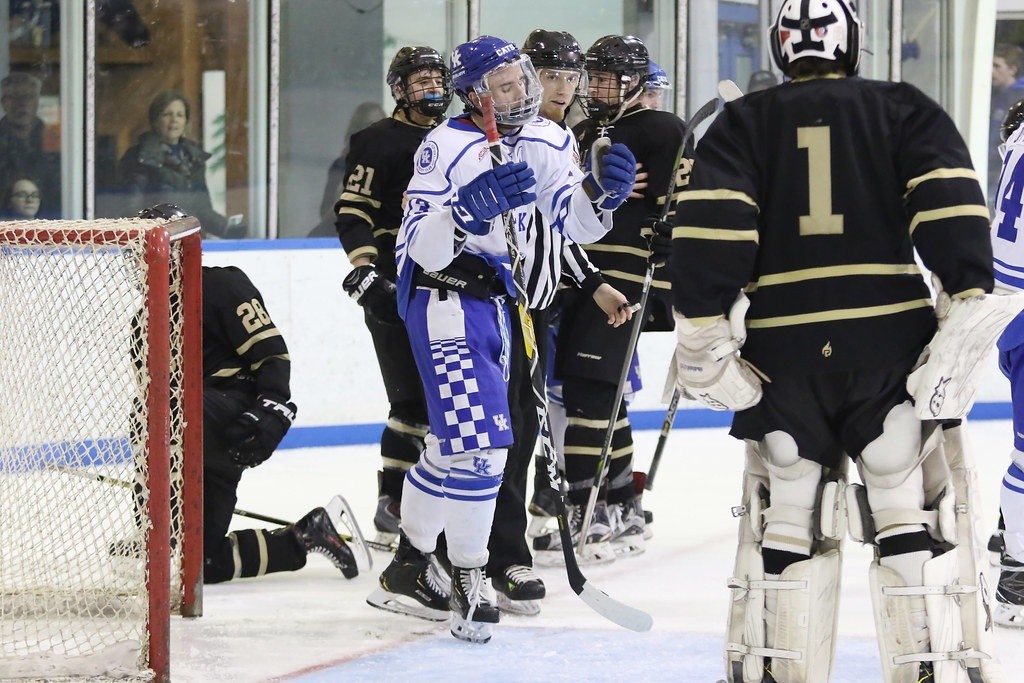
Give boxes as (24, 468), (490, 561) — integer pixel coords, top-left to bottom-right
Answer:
(450, 160), (539, 235)
(342, 263), (403, 327)
(642, 214), (674, 266)
(581, 135), (637, 212)
(228, 393), (297, 467)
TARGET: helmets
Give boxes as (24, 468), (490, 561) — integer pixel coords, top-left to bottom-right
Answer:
(448, 33), (544, 127)
(766, 0), (863, 79)
(643, 60), (672, 91)
(122, 201), (188, 294)
(386, 46), (456, 117)
(576, 34), (648, 119)
(519, 28), (586, 90)
(999, 98), (1024, 142)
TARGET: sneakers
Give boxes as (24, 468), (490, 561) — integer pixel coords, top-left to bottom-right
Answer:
(367, 528), (454, 622)
(993, 532), (1024, 629)
(371, 466), (406, 547)
(111, 534), (180, 583)
(487, 454), (653, 614)
(448, 563), (499, 644)
(985, 507), (1005, 566)
(279, 495), (373, 580)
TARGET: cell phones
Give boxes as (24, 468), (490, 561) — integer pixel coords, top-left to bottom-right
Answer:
(223, 213), (244, 237)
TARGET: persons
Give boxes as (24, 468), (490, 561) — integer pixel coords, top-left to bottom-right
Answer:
(309, 31), (696, 647)
(106, 201), (373, 583)
(657, 0), (1024, 683)
(1, 72), (61, 218)
(109, 87), (249, 239)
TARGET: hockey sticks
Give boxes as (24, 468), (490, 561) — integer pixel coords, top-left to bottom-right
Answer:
(575, 97), (721, 557)
(41, 461), (399, 554)
(480, 87), (657, 634)
(642, 77), (744, 493)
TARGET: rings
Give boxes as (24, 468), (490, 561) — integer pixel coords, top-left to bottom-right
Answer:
(618, 306), (623, 313)
(623, 301), (631, 308)
